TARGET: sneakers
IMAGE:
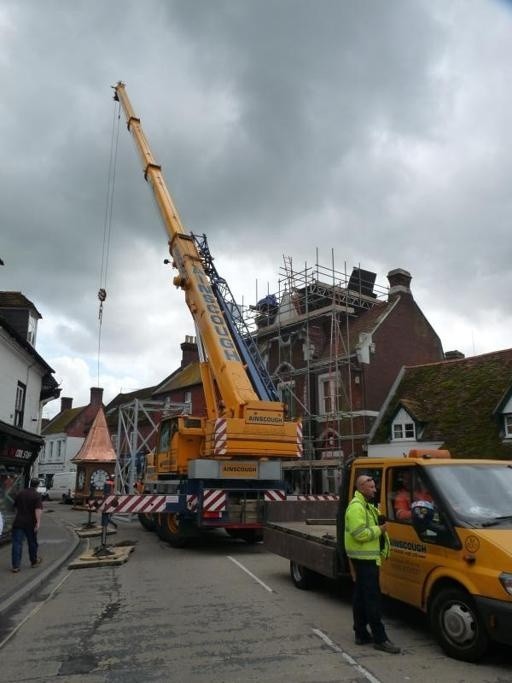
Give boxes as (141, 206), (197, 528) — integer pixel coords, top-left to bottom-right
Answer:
(32, 558), (42, 568)
(12, 567), (19, 573)
(355, 637), (372, 645)
(373, 641), (400, 653)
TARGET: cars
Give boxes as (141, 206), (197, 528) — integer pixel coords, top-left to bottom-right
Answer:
(35, 478), (48, 497)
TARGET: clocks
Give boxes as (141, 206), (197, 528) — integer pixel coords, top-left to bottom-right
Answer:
(77, 468), (112, 492)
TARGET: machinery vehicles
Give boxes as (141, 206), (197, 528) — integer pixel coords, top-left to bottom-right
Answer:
(84, 80), (319, 557)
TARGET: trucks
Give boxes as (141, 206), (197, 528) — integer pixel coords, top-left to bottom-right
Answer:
(262, 447), (512, 662)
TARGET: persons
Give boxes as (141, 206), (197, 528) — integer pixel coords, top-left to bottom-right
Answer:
(10, 477), (42, 572)
(344, 475), (401, 653)
(133, 479), (143, 493)
(394, 472), (434, 518)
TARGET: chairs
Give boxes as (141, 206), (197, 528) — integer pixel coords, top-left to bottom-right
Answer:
(388, 492), (400, 521)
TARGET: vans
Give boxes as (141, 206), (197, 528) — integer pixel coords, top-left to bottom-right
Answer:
(47, 471), (77, 504)
